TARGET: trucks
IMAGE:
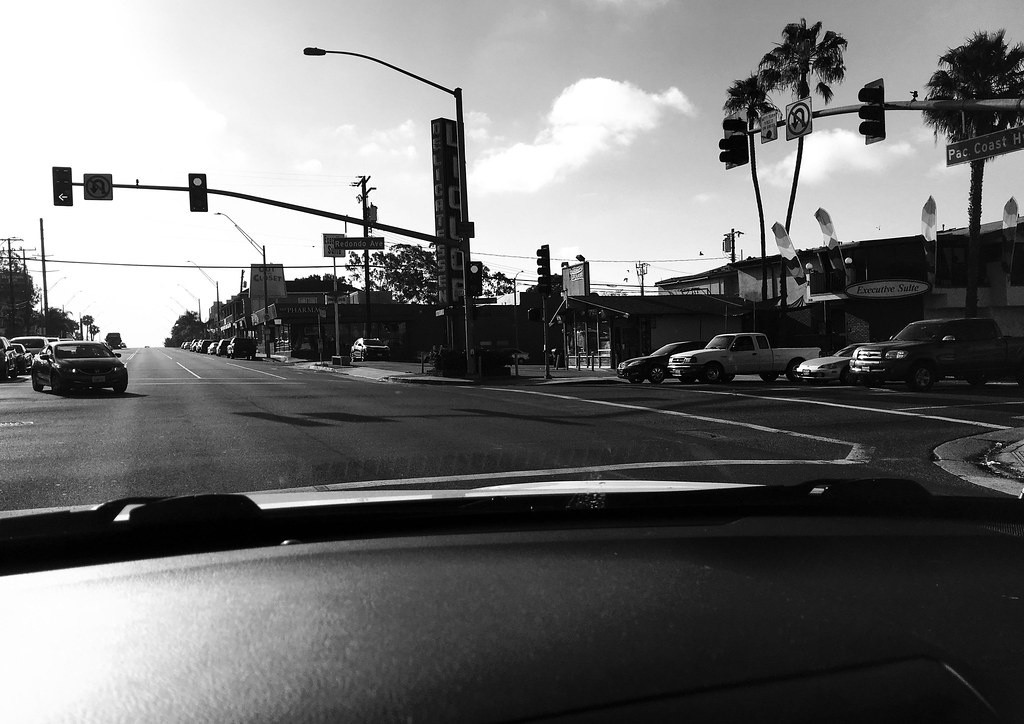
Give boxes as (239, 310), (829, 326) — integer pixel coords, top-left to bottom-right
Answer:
(105, 333), (122, 348)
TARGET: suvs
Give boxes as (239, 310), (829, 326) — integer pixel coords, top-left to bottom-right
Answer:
(350, 337), (390, 362)
(227, 337), (257, 360)
(215, 339), (230, 357)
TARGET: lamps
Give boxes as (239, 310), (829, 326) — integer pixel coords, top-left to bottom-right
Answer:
(845, 257), (856, 268)
(805, 263), (817, 274)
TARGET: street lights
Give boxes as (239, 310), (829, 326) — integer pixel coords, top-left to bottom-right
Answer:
(302, 46), (479, 375)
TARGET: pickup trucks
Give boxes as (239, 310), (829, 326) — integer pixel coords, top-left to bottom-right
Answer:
(849, 318), (1023, 390)
(667, 331), (824, 385)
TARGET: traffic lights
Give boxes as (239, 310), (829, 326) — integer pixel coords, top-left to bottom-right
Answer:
(52, 167), (73, 206)
(469, 261), (483, 295)
(189, 174), (208, 212)
(536, 244), (553, 296)
(858, 78), (885, 144)
(718, 108), (750, 169)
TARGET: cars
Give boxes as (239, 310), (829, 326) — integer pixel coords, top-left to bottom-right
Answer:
(502, 347), (530, 365)
(0, 334), (75, 382)
(31, 340), (129, 394)
(180, 339), (212, 354)
(618, 340), (711, 385)
(793, 341), (873, 386)
(208, 342), (219, 355)
